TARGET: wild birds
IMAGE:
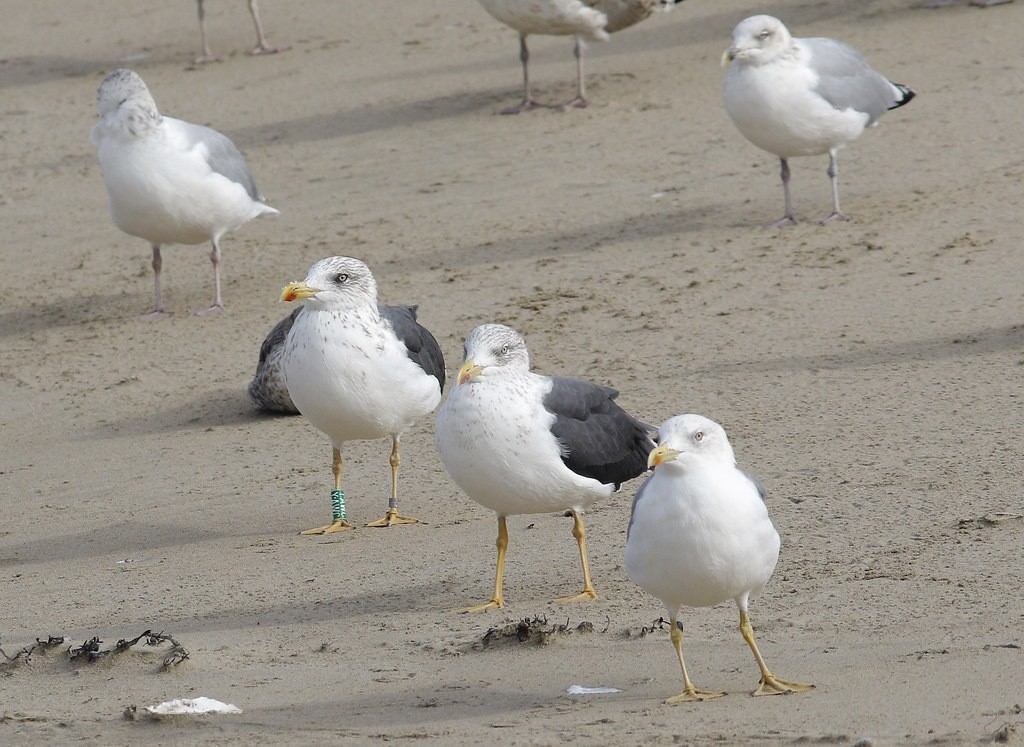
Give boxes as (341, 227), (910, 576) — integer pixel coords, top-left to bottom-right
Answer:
(433, 323), (664, 616)
(247, 254), (448, 537)
(622, 412), (817, 706)
(93, 69), (284, 315)
(185, 1), (690, 117)
(719, 13), (917, 228)
